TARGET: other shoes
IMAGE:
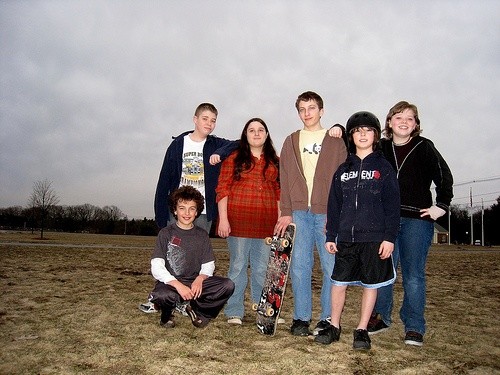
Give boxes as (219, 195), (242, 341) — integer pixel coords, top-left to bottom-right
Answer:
(228, 315), (242, 324)
(277, 317), (285, 323)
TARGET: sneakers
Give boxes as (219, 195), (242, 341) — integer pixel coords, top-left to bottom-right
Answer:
(353, 329), (371, 350)
(312, 317), (331, 335)
(139, 301), (158, 313)
(314, 325), (341, 344)
(366, 313), (389, 335)
(185, 304), (206, 327)
(160, 308), (176, 327)
(404, 330), (424, 345)
(291, 318), (311, 335)
(176, 303), (189, 316)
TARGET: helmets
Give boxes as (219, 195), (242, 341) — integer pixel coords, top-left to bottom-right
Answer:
(346, 111), (381, 146)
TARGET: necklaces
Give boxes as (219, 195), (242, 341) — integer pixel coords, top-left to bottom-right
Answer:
(393, 136), (411, 145)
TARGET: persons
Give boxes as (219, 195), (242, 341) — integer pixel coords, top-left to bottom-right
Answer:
(150, 185), (236, 329)
(141, 102), (241, 316)
(274, 91), (348, 337)
(327, 101), (454, 346)
(314, 110), (401, 354)
(215, 118), (285, 326)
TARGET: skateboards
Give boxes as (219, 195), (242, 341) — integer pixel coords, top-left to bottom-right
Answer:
(251, 222), (297, 337)
